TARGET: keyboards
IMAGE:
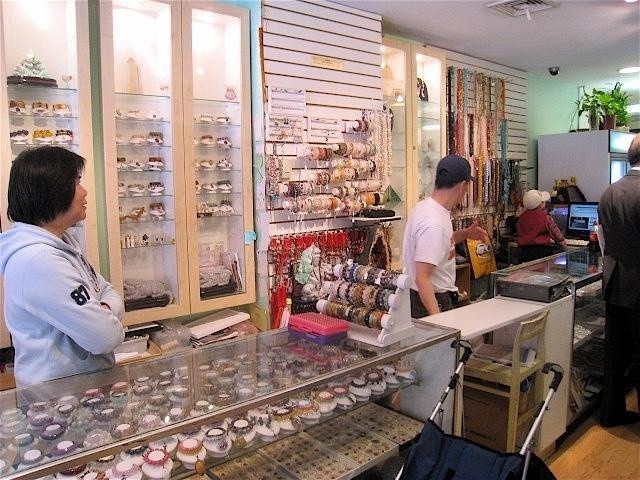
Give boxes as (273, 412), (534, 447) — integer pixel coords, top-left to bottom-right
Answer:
(565, 239), (590, 246)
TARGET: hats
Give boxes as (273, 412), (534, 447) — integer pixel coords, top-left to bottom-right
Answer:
(437, 154), (477, 182)
(523, 190), (551, 210)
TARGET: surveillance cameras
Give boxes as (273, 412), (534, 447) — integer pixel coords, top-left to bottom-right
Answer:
(549, 67), (559, 75)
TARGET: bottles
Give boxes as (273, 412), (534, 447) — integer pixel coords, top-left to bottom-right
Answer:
(587, 220), (600, 253)
(588, 255), (598, 273)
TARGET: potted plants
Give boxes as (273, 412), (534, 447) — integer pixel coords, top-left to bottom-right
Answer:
(572, 79), (630, 130)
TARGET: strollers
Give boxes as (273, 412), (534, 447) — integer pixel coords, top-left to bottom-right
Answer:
(389, 337), (566, 480)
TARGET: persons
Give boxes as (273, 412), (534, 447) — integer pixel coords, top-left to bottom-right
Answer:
(595, 133), (640, 429)
(1, 143), (126, 391)
(402, 156), (491, 323)
(513, 188), (568, 261)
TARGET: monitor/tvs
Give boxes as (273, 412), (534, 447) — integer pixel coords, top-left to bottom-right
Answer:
(567, 202), (600, 236)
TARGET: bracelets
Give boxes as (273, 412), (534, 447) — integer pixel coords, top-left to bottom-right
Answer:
(2, 338), (414, 480)
(10, 99), (27, 109)
(33, 101), (49, 110)
(33, 130), (53, 140)
(266, 110), (401, 329)
(10, 129), (28, 139)
(55, 129), (72, 137)
(115, 109), (234, 247)
(52, 104), (71, 111)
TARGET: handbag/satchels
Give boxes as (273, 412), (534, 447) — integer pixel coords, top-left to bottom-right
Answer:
(462, 230), (497, 280)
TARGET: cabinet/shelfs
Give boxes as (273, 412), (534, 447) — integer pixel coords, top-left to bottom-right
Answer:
(3, 0), (256, 351)
(0, 307), (459, 480)
(379, 35), (448, 275)
(494, 247), (605, 432)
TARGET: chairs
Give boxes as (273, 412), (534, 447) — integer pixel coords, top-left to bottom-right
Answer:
(455, 308), (545, 458)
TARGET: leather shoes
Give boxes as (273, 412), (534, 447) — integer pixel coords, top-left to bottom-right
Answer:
(602, 410), (638, 426)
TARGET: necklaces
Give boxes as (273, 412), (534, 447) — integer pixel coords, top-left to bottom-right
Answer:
(450, 65), (522, 239)
